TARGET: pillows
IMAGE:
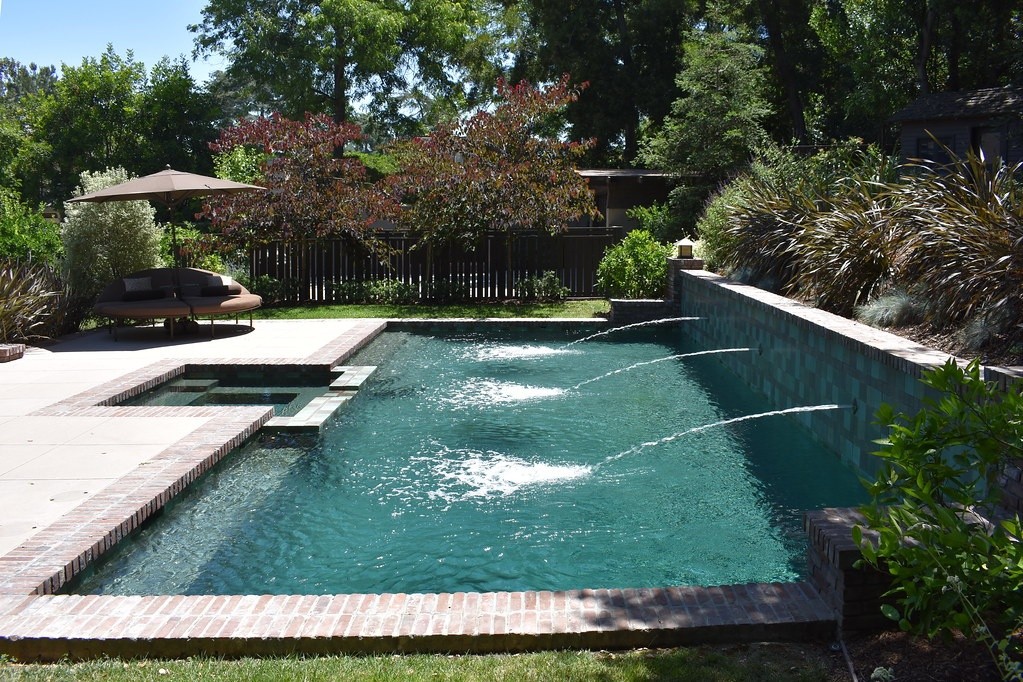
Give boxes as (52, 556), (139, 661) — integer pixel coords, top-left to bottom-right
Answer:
(203, 276), (233, 286)
(120, 290), (166, 302)
(200, 285), (228, 297)
(228, 284), (242, 295)
(122, 277), (152, 292)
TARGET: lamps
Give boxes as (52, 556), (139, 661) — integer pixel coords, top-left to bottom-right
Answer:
(676, 237), (695, 259)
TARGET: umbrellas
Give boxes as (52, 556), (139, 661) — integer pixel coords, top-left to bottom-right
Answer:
(66, 165), (268, 300)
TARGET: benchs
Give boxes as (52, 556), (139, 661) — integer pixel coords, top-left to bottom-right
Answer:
(174, 267), (263, 338)
(93, 267), (192, 342)
